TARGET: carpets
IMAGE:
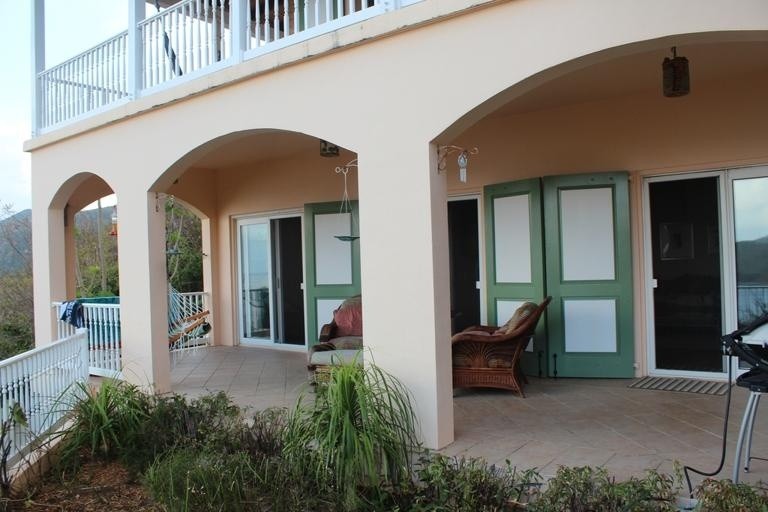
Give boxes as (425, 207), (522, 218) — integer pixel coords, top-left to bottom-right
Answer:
(627, 374), (735, 398)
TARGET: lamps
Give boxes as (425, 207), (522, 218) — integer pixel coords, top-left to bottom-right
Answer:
(437, 143), (479, 185)
(107, 203), (117, 238)
(659, 46), (693, 100)
(318, 139), (340, 158)
(155, 190), (177, 212)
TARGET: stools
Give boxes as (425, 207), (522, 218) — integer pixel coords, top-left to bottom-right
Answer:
(307, 348), (369, 394)
(733, 362), (768, 494)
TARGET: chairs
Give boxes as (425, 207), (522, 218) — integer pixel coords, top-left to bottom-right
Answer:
(447, 295), (555, 399)
(305, 290), (367, 386)
